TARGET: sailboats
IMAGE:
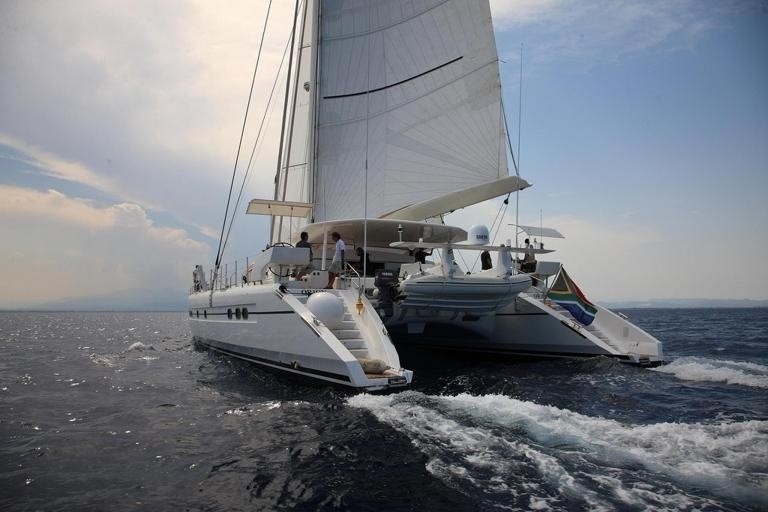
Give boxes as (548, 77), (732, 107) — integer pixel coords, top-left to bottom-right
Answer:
(187, 0), (666, 394)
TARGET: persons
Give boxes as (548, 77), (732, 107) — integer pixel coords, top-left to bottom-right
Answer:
(295, 231), (313, 281)
(322, 231), (345, 290)
(355, 247), (371, 277)
(519, 236), (537, 272)
(192, 264), (203, 292)
(480, 247), (494, 271)
(413, 247), (434, 264)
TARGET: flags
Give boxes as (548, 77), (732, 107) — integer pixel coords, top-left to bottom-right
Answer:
(547, 266), (598, 327)
